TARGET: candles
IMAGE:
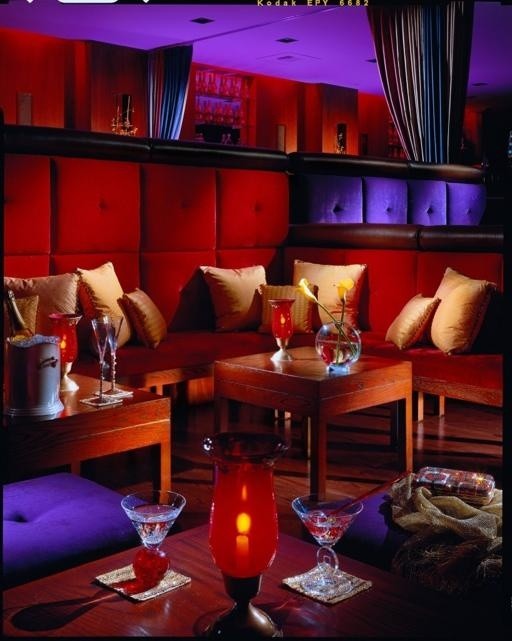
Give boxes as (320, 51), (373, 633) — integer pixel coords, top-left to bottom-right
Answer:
(233, 512), (252, 573)
(279, 314), (285, 339)
(61, 341), (66, 362)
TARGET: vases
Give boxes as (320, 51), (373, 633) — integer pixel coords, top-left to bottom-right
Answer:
(314, 319), (363, 374)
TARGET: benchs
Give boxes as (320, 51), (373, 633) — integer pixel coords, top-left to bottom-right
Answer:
(0, 330), (276, 409)
(283, 245), (503, 424)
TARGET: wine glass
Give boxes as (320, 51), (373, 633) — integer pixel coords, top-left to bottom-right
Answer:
(194, 71), (242, 127)
(198, 430), (287, 640)
(290, 492), (363, 597)
(267, 298), (297, 362)
(120, 489), (187, 586)
(90, 315), (124, 404)
(45, 314), (85, 393)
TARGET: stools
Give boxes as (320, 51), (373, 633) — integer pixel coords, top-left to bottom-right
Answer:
(2, 469), (183, 591)
(306, 490), (414, 572)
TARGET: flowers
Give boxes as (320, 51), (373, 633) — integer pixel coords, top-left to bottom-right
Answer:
(291, 277), (358, 364)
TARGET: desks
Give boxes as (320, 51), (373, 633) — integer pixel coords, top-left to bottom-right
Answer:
(212, 343), (415, 506)
(3, 370), (174, 503)
(1, 519), (503, 641)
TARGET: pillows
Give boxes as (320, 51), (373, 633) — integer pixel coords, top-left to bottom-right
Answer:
(293, 257), (368, 339)
(422, 264), (497, 356)
(197, 263), (267, 332)
(385, 291), (440, 350)
(73, 260), (134, 352)
(118, 286), (169, 350)
(256, 282), (319, 335)
(2, 273), (81, 337)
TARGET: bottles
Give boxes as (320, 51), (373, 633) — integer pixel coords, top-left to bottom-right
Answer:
(5, 290), (35, 341)
